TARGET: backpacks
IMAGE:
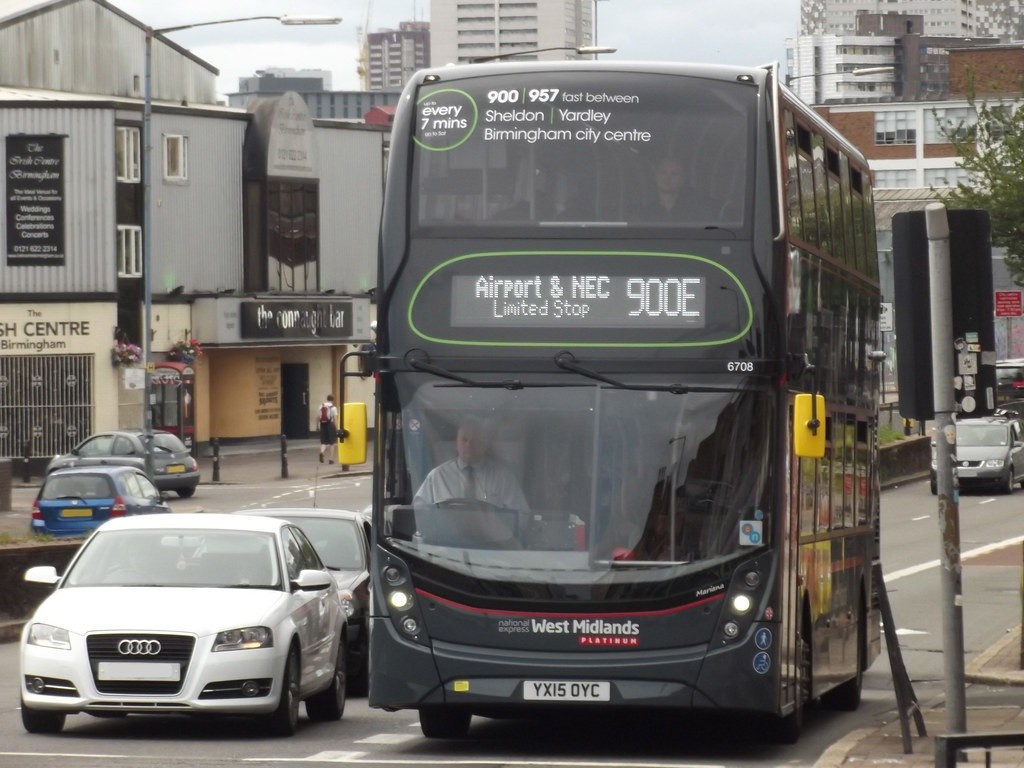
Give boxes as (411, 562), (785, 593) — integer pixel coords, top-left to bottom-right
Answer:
(319, 401), (334, 423)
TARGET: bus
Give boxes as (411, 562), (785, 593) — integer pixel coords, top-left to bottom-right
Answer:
(334, 58), (885, 740)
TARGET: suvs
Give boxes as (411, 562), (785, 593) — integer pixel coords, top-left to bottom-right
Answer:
(994, 357), (1024, 404)
(44, 430), (201, 500)
(930, 416), (1024, 496)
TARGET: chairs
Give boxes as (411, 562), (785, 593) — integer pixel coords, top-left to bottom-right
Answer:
(99, 479), (127, 498)
(54, 478), (83, 497)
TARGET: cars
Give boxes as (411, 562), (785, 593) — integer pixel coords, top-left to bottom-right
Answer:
(32, 468), (170, 538)
(238, 506), (373, 689)
(17, 514), (352, 738)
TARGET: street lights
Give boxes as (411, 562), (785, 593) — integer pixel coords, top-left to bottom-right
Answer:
(139, 12), (345, 429)
(785, 65), (895, 84)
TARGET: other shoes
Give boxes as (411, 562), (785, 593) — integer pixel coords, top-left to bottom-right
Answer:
(329, 460), (335, 464)
(319, 452), (324, 463)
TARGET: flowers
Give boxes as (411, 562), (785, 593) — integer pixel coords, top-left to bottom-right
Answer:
(112, 332), (144, 366)
(170, 338), (204, 364)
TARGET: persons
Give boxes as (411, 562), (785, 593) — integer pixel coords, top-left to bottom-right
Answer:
(412, 421), (530, 529)
(316, 395), (338, 464)
(629, 157), (703, 223)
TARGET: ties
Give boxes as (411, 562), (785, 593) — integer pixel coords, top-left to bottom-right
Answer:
(462, 466), (477, 499)
